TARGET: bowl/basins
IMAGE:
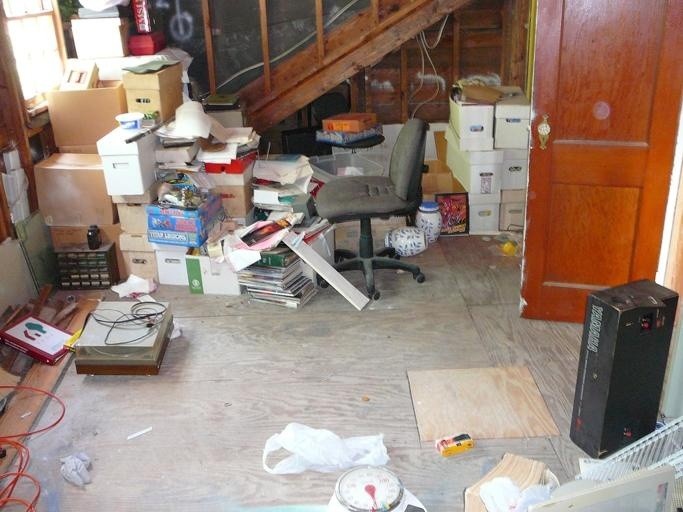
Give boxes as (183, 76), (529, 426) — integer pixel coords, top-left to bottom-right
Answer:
(115, 112), (147, 129)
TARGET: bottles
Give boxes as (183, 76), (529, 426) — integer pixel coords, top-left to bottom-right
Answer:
(87, 224), (103, 250)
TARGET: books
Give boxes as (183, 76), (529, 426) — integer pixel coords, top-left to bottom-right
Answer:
(152, 100), (315, 206)
(60, 58), (103, 91)
(240, 246), (322, 309)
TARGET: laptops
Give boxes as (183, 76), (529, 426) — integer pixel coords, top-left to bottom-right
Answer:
(202, 94), (240, 109)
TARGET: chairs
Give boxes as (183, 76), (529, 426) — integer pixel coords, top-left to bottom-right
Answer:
(309, 89), (386, 151)
(312, 116), (436, 304)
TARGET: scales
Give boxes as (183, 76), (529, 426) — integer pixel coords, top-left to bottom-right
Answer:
(201, 92), (243, 111)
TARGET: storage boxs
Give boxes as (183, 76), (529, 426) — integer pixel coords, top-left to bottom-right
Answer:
(33, 13), (259, 297)
(306, 153), (383, 184)
(420, 82), (528, 236)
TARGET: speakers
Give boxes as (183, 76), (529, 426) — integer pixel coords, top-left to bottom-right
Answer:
(570, 278), (679, 460)
